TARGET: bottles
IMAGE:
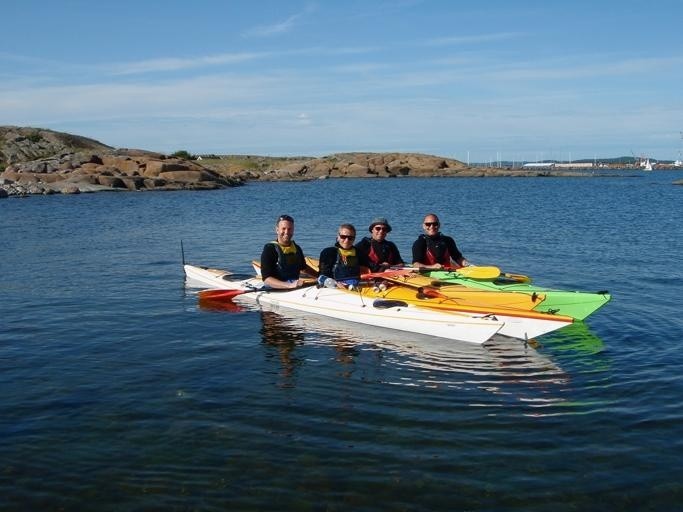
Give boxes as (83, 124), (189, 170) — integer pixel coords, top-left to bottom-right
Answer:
(317, 274), (337, 288)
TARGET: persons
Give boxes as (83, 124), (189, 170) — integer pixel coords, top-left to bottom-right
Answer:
(260, 213), (319, 290)
(354, 218), (404, 273)
(411, 213), (469, 270)
(316, 223), (360, 288)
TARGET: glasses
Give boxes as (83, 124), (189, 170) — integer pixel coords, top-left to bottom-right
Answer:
(376, 226), (388, 231)
(279, 215), (293, 221)
(338, 232), (355, 241)
(423, 221), (439, 226)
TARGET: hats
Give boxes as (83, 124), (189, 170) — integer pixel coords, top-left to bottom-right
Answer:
(369, 217), (392, 233)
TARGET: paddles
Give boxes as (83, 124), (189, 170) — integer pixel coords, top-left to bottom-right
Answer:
(501, 271), (530, 286)
(407, 266), (500, 279)
(196, 268), (415, 301)
(382, 274), (448, 299)
